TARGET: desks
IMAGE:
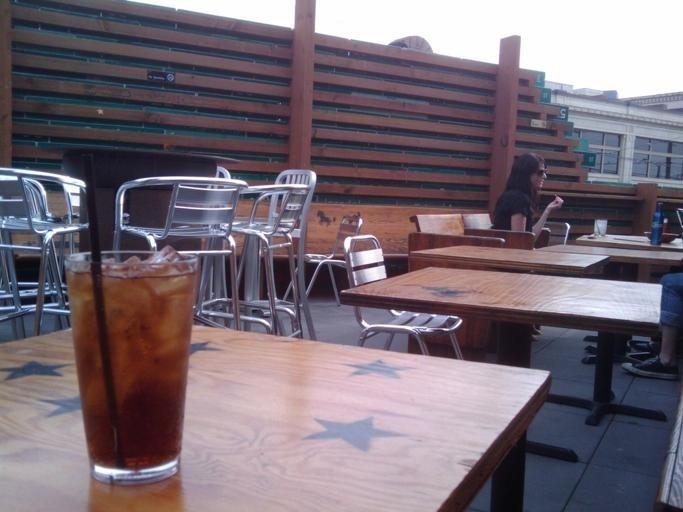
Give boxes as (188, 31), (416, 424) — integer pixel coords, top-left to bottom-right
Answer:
(537, 244), (683, 281)
(409, 245), (610, 277)
(61, 147), (242, 253)
(340, 266), (667, 462)
(575, 234), (683, 281)
(0, 324), (553, 512)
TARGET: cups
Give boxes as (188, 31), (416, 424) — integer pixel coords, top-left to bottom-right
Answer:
(63, 248), (197, 485)
(593, 219), (608, 239)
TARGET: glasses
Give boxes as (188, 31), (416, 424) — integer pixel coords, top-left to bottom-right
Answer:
(532, 168), (548, 176)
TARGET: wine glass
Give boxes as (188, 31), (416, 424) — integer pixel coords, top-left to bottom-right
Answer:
(675, 207), (683, 228)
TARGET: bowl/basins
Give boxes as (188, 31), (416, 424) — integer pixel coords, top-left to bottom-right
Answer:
(644, 231), (678, 243)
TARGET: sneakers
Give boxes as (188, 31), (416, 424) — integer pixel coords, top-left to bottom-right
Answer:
(622, 337), (678, 380)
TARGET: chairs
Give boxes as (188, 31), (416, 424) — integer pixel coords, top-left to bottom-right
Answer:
(343, 234), (463, 360)
(462, 209), (551, 250)
(281, 215), (364, 307)
(0, 165), (319, 343)
(407, 214), (536, 354)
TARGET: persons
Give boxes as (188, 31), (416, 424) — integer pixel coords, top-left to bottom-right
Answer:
(490, 151), (564, 342)
(621, 270), (683, 380)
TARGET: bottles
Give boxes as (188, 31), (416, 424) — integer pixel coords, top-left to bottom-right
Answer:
(651, 200), (664, 247)
